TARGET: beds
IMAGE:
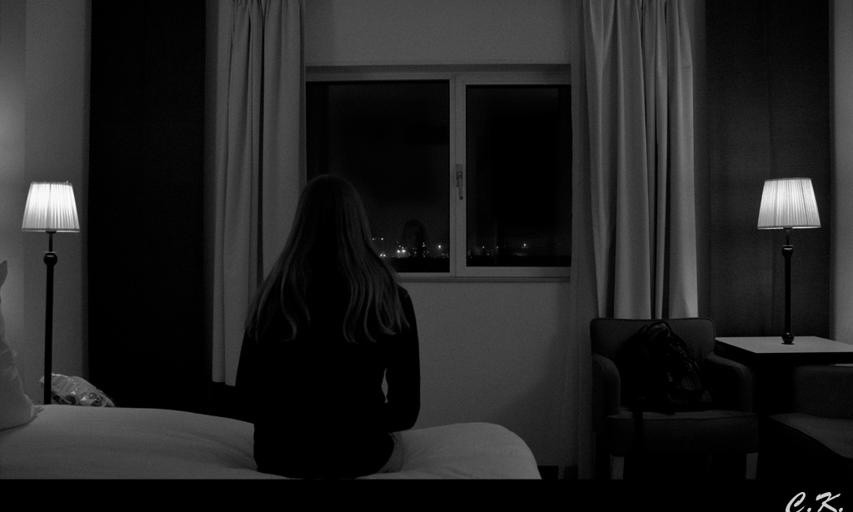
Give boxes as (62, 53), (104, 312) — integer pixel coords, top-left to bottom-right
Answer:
(0, 403), (543, 480)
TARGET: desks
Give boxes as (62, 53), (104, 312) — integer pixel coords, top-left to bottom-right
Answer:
(713, 334), (853, 371)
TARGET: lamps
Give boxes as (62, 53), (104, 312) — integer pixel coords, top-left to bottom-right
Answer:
(757, 178), (822, 345)
(21, 180), (82, 405)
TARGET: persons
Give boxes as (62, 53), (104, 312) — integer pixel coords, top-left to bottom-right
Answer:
(236, 174), (420, 478)
(395, 218), (430, 270)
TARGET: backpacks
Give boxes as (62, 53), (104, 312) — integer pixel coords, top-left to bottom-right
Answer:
(614, 321), (703, 415)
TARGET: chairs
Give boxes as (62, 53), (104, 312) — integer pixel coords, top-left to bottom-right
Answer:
(588, 316), (759, 482)
